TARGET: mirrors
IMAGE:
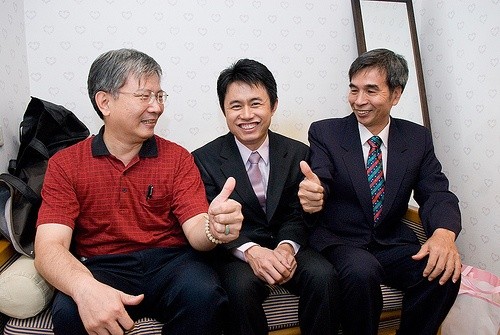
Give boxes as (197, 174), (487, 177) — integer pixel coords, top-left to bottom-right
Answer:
(351, 0), (433, 138)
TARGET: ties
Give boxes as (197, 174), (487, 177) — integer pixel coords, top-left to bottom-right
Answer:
(247, 154), (266, 212)
(366, 136), (385, 227)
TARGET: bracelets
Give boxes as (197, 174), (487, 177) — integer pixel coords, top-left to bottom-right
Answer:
(205, 216), (229, 244)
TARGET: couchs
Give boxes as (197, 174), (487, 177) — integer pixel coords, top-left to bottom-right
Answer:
(0, 205), (441, 335)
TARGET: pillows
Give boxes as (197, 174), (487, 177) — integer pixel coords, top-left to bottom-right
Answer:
(0, 256), (58, 318)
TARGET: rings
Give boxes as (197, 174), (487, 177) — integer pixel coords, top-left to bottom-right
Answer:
(225, 224), (230, 235)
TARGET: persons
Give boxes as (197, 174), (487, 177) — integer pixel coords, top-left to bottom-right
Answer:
(189, 58), (343, 335)
(297, 48), (463, 335)
(34, 47), (245, 335)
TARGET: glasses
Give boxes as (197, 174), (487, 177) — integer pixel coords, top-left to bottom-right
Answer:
(113, 88), (168, 105)
